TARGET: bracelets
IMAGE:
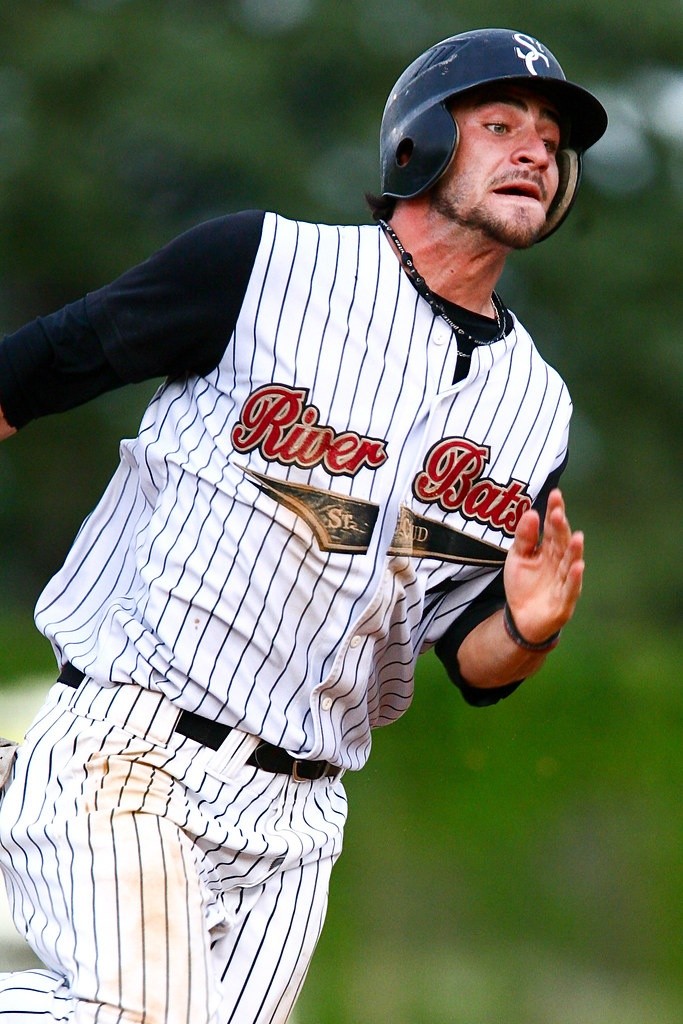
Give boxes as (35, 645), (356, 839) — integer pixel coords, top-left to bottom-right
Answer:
(501, 602), (562, 651)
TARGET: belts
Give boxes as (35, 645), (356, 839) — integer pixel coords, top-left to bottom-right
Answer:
(56, 663), (342, 784)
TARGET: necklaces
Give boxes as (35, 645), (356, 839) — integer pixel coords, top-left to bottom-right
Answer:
(380, 218), (506, 344)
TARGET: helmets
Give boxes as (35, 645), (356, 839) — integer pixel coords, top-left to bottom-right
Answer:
(379, 28), (609, 243)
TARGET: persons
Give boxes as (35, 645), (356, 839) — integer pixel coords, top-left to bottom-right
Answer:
(0, 28), (611, 1023)
